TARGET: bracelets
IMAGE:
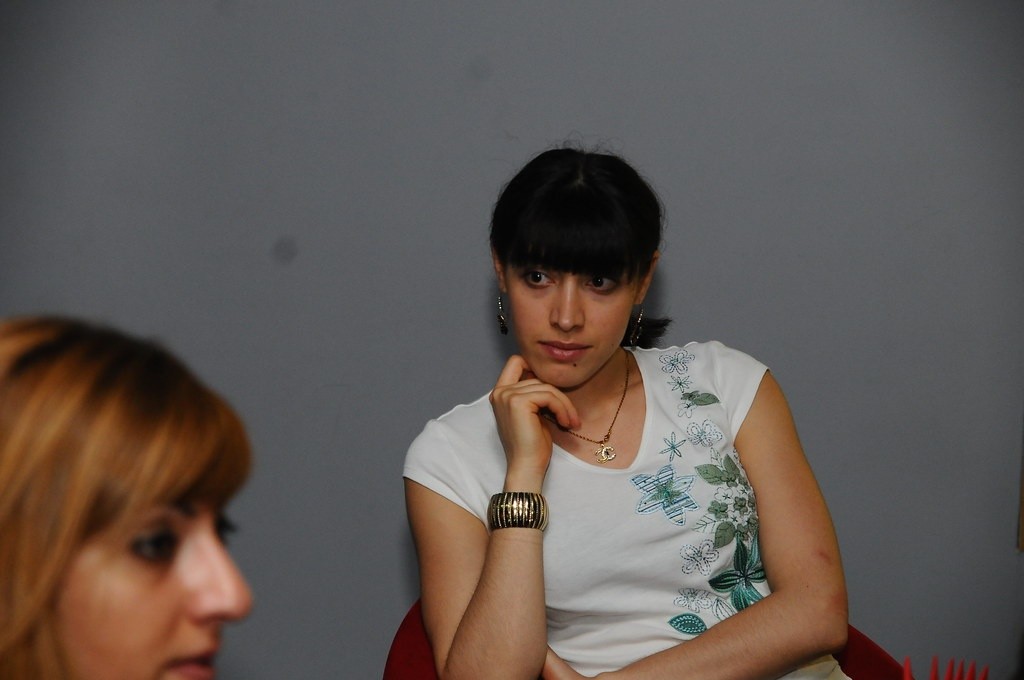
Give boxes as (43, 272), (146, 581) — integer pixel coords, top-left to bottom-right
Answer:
(487, 491), (549, 531)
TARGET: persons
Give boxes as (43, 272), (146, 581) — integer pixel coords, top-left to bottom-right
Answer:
(403, 151), (851, 680)
(1, 314), (253, 680)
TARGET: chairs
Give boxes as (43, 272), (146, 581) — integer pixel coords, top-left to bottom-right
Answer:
(384, 599), (915, 680)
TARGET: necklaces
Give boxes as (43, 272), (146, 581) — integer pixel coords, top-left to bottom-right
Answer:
(539, 348), (630, 462)
